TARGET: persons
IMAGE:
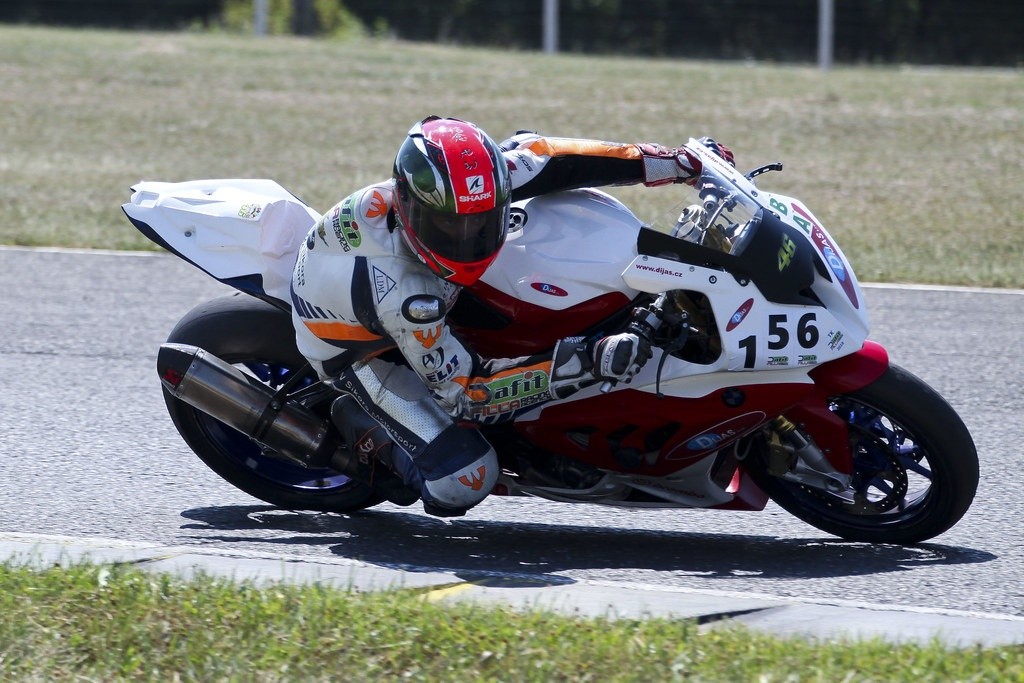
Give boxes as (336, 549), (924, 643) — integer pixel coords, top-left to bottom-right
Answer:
(291, 117), (736, 523)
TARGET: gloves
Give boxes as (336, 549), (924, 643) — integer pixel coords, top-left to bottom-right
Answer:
(634, 136), (737, 188)
(549, 332), (652, 399)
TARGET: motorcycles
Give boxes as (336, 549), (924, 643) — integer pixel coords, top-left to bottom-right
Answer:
(119, 134), (981, 546)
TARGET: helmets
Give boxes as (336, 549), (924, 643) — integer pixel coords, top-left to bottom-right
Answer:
(392, 116), (511, 288)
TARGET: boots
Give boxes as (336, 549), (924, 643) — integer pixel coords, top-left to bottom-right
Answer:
(331, 394), (393, 481)
